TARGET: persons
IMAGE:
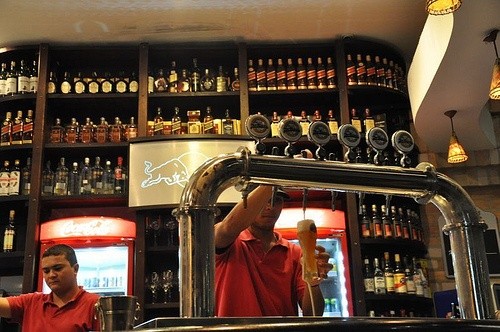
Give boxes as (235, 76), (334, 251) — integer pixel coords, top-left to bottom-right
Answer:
(0, 244), (101, 332)
(214, 182), (333, 316)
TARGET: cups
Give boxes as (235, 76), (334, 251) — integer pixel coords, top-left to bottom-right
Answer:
(296, 219), (318, 283)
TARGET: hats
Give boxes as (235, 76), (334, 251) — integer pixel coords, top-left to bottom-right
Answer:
(242, 182), (291, 202)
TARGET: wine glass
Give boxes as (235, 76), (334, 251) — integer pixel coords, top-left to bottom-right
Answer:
(145, 213), (176, 247)
(147, 269), (178, 304)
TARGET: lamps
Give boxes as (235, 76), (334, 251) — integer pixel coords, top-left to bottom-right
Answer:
(445, 110), (468, 163)
(425, 0), (460, 15)
(483, 30), (500, 100)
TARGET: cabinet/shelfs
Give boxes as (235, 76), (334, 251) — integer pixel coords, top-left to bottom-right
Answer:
(0, 35), (437, 325)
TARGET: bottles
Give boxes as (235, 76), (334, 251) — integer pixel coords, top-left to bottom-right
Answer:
(51, 117), (137, 144)
(47, 70), (139, 93)
(369, 309), (415, 318)
(223, 108), (234, 135)
(153, 107), (164, 136)
(0, 59), (38, 98)
(148, 57), (240, 93)
(42, 157), (126, 194)
(0, 157), (32, 196)
(248, 54), (410, 92)
(171, 106), (182, 135)
(257, 108), (375, 138)
(358, 205), (425, 244)
(362, 251), (432, 299)
(203, 107), (215, 134)
(2, 209), (17, 255)
(0, 110), (34, 147)
(93, 295), (138, 332)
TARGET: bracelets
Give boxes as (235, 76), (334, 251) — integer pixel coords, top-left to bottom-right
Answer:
(311, 284), (318, 287)
